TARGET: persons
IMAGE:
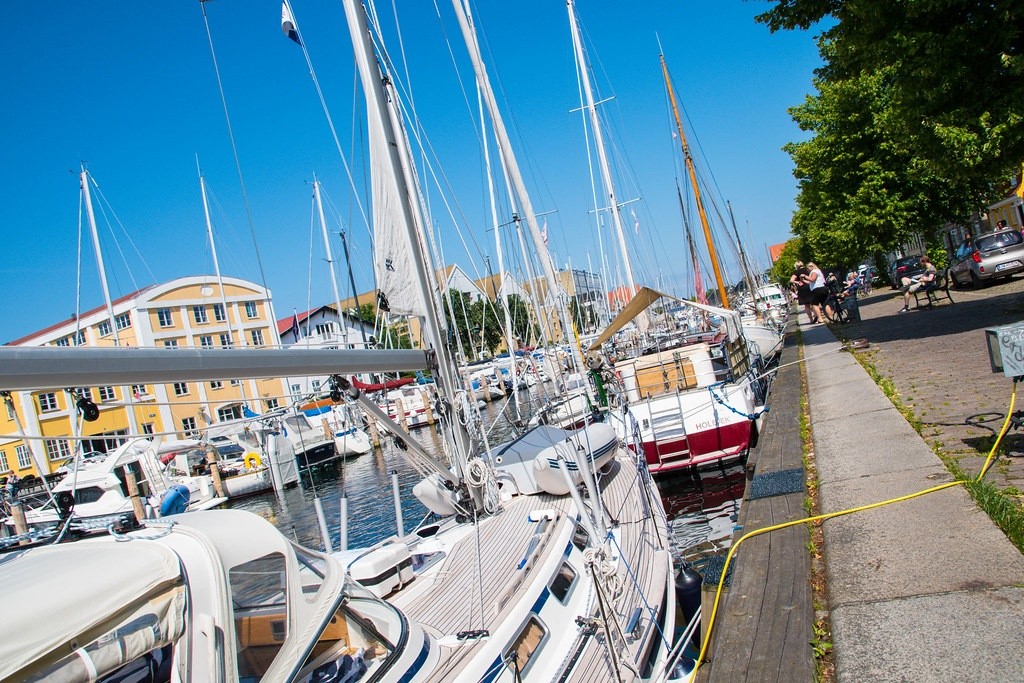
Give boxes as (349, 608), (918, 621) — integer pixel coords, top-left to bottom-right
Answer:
(965, 234), (971, 247)
(897, 256), (937, 314)
(789, 261), (858, 323)
(1020, 226), (1024, 237)
(993, 220), (1013, 242)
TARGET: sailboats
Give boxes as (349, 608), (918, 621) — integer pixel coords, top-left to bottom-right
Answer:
(1, 0), (792, 682)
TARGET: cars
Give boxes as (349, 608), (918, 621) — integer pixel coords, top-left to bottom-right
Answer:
(889, 254), (927, 290)
(948, 227), (1024, 288)
(856, 264), (885, 289)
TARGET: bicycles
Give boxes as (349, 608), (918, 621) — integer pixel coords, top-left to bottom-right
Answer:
(820, 278), (851, 325)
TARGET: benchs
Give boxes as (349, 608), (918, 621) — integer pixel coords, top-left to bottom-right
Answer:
(914, 267), (954, 310)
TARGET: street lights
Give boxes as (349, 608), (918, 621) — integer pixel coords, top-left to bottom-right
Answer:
(333, 229), (376, 385)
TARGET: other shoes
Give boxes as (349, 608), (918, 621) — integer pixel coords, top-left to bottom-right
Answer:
(814, 320), (824, 326)
(810, 316), (818, 324)
(900, 307), (910, 313)
(829, 321), (834, 326)
(899, 285), (910, 291)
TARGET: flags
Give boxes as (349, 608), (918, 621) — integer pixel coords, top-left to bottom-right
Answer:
(281, 2), (304, 46)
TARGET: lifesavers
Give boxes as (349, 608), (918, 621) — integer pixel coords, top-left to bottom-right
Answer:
(245, 453), (261, 469)
